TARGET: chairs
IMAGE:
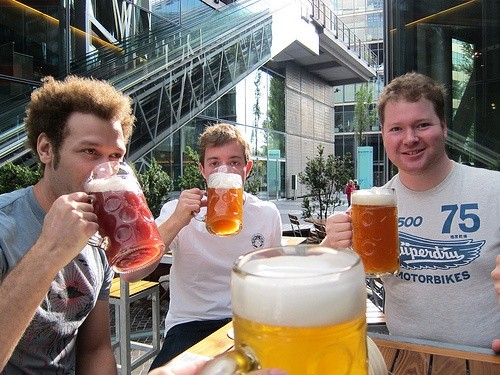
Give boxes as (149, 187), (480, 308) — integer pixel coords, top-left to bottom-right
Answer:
(288, 214), (302, 237)
(314, 221), (326, 244)
(108, 269), (159, 375)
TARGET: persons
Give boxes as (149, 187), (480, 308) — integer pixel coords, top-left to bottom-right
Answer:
(0, 73), (137, 375)
(319, 70), (500, 353)
(147, 122), (283, 373)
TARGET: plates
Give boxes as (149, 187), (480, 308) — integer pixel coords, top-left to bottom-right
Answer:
(367, 335), (387, 375)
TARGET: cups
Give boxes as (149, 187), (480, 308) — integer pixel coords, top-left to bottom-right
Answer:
(192, 165), (243, 237)
(345, 188), (399, 279)
(86, 161), (166, 274)
(198, 244), (370, 375)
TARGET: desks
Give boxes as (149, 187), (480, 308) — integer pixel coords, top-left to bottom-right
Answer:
(158, 236), (308, 265)
(165, 320), (500, 375)
(304, 219), (327, 225)
